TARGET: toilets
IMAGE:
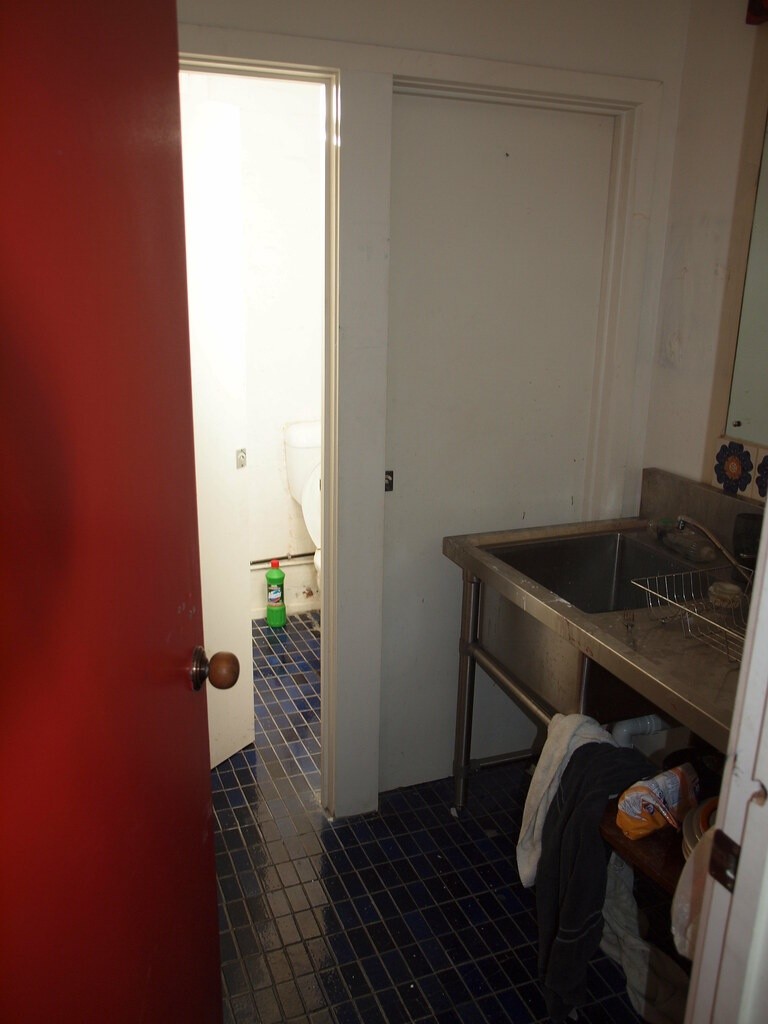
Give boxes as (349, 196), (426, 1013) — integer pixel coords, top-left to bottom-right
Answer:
(283, 418), (321, 593)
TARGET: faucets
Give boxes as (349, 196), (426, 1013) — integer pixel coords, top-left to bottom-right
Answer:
(678, 507), (757, 588)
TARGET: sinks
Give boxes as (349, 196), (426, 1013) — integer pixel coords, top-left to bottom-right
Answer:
(478, 519), (723, 622)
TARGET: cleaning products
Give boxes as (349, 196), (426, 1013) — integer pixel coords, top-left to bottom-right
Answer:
(262, 557), (289, 628)
(614, 759), (704, 845)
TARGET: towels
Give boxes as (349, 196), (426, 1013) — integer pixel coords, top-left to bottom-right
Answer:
(515, 710), (616, 895)
(601, 848), (693, 1023)
(538, 738), (659, 1024)
(668, 820), (716, 963)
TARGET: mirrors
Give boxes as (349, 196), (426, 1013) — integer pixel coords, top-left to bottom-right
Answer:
(705, 20), (768, 459)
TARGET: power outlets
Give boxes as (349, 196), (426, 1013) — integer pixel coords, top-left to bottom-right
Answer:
(236, 448), (247, 469)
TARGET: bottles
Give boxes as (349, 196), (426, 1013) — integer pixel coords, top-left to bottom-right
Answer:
(265, 560), (287, 628)
